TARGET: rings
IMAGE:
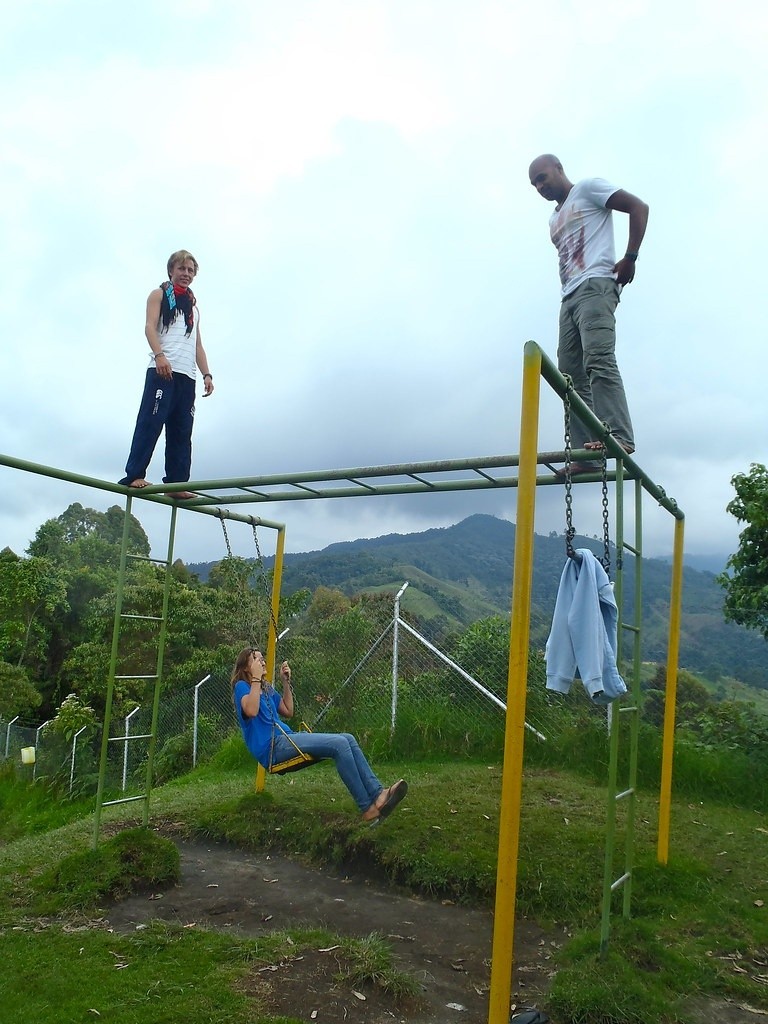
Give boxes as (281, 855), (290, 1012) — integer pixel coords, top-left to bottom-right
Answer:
(167, 368), (171, 371)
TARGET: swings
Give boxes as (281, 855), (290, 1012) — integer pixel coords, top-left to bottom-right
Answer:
(215, 517), (319, 776)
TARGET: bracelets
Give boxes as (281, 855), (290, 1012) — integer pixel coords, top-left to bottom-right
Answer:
(153, 353), (164, 360)
(203, 374), (213, 380)
(250, 681), (261, 683)
(251, 676), (261, 680)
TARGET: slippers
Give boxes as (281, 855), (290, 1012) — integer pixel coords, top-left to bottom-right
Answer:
(378, 781), (408, 818)
(369, 816), (387, 830)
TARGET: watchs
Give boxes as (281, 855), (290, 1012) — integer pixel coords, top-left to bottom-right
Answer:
(624, 252), (638, 260)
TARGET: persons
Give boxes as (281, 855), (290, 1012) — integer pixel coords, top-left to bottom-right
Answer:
(528, 154), (652, 474)
(118, 249), (214, 498)
(230, 645), (409, 831)
